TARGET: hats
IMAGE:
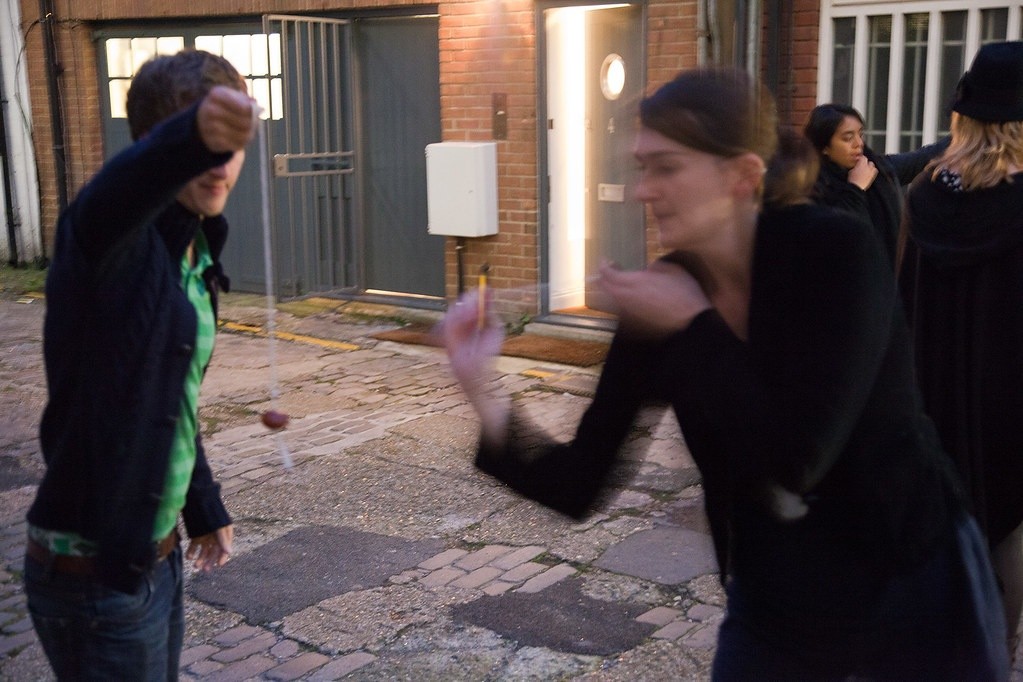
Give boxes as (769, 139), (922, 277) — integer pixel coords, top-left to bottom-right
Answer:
(951, 42), (1023, 121)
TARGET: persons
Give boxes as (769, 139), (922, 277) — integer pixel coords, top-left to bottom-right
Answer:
(442, 37), (1023, 682)
(20, 49), (263, 682)
